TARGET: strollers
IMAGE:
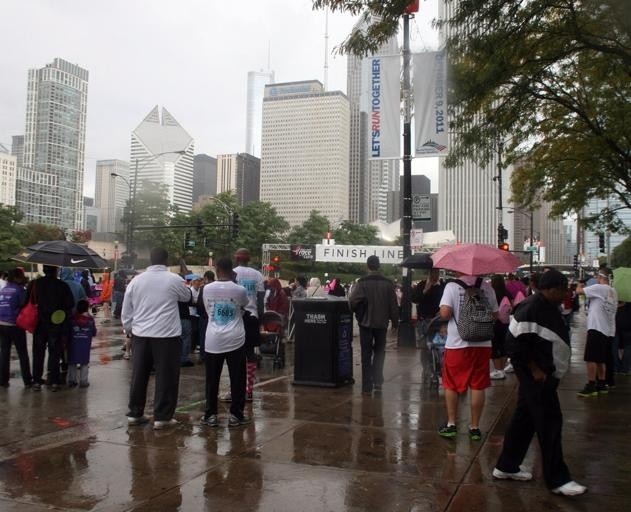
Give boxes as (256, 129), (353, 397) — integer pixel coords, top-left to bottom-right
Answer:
(257, 312), (284, 368)
(418, 313), (450, 390)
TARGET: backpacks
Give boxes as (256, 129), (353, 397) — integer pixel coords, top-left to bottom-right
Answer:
(497, 296), (512, 325)
(511, 282), (526, 307)
(445, 275), (497, 343)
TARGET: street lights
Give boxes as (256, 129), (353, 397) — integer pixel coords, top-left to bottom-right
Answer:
(114, 240), (119, 272)
(496, 205), (534, 272)
(129, 149), (187, 255)
(111, 172), (133, 258)
(209, 251), (214, 271)
(599, 233), (605, 248)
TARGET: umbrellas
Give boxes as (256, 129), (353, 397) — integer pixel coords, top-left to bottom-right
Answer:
(608, 267), (631, 304)
(10, 241), (111, 270)
(395, 253), (437, 274)
(430, 243), (525, 277)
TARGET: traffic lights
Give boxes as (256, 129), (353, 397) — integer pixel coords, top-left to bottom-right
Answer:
(600, 248), (605, 253)
(502, 243), (509, 251)
(498, 226), (504, 249)
(233, 213), (240, 228)
(274, 256), (279, 262)
(184, 230), (191, 248)
(574, 255), (578, 267)
(197, 216), (203, 234)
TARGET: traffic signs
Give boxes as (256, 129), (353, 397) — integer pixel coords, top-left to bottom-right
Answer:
(527, 246), (538, 250)
(411, 192), (433, 223)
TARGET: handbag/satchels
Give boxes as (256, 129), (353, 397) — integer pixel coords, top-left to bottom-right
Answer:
(16, 304), (39, 333)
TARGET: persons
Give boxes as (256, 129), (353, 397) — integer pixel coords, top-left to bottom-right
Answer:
(493, 270), (588, 498)
(0, 264), (125, 390)
(120, 247), (192, 431)
(182, 247), (346, 427)
(398, 263), (631, 440)
(348, 255), (399, 396)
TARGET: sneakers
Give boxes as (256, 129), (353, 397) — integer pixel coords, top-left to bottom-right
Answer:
(468, 424), (481, 439)
(489, 364), (515, 379)
(201, 414), (217, 427)
(120, 345), (132, 359)
(552, 481), (588, 498)
(179, 360), (194, 367)
(577, 379), (616, 397)
(152, 419), (178, 430)
(23, 365), (90, 392)
(229, 415), (251, 428)
(438, 424), (456, 437)
(193, 345), (201, 354)
(361, 382), (382, 396)
(127, 414), (150, 426)
(492, 467), (533, 482)
(219, 389), (252, 402)
(1, 380), (10, 388)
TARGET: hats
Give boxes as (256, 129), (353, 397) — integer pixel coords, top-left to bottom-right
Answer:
(192, 275), (201, 280)
